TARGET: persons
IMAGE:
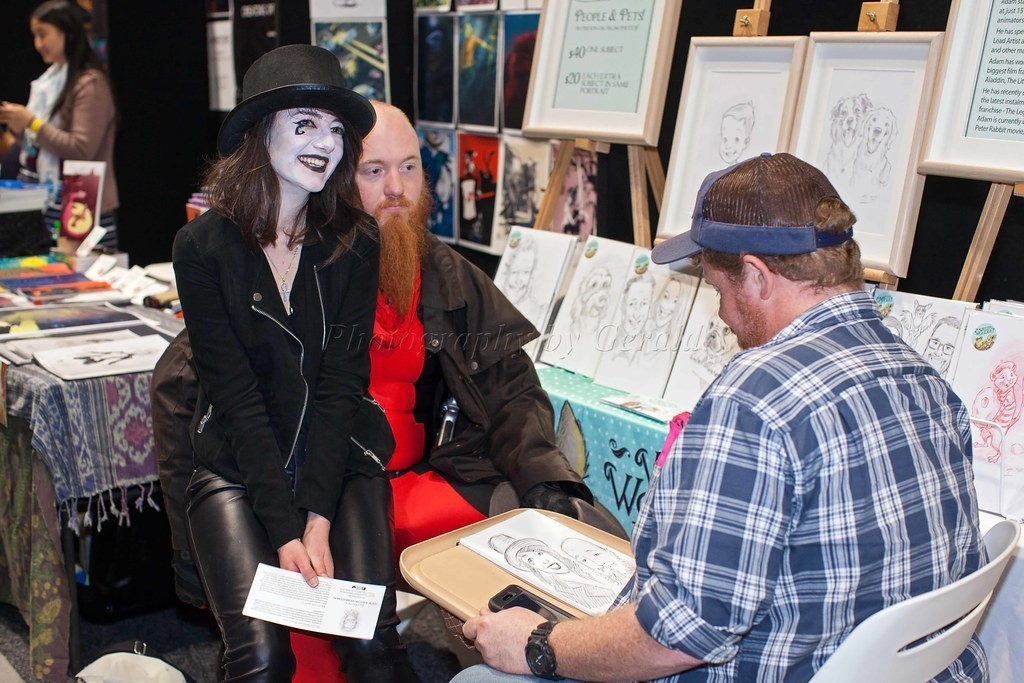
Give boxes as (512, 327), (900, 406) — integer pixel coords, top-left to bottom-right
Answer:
(462, 153), (993, 683)
(171, 43), (419, 683)
(0, 0), (119, 254)
(150, 102), (593, 683)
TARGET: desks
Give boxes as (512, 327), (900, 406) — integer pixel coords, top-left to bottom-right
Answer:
(0, 253), (221, 683)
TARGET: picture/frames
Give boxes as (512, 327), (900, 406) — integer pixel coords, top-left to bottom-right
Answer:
(789, 29), (946, 279)
(521, 0), (682, 148)
(653, 36), (808, 242)
(918, 0), (1024, 185)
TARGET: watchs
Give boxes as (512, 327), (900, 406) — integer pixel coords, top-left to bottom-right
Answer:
(525, 621), (564, 681)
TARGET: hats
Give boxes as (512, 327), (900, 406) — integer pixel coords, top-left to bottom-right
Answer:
(652, 152), (854, 265)
(216, 45), (376, 159)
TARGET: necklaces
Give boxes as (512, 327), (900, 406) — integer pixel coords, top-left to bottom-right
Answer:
(261, 244), (298, 303)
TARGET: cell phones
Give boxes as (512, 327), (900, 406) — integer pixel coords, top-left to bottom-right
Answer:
(488, 584), (580, 622)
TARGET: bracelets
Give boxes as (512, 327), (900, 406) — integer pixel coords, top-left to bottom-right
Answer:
(31, 119), (43, 133)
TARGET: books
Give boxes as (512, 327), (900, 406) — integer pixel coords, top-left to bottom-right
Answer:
(0, 256), (179, 382)
(186, 171), (226, 224)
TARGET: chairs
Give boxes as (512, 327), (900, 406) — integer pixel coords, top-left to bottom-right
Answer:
(809, 521), (1021, 683)
